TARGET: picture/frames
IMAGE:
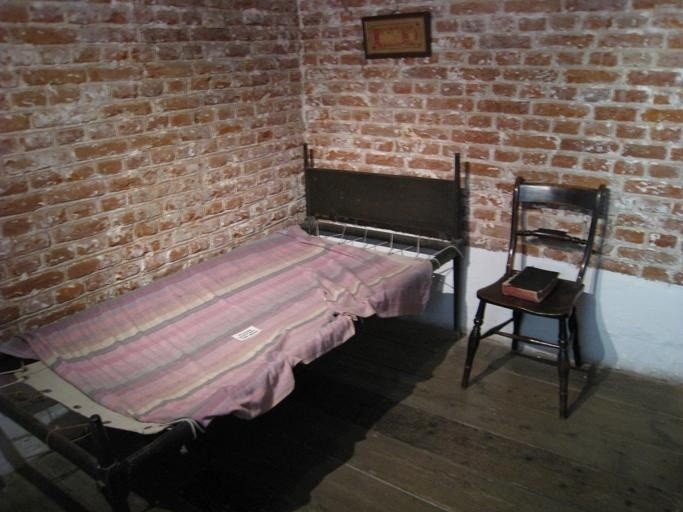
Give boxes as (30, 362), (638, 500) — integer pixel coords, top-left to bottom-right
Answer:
(362, 12), (431, 59)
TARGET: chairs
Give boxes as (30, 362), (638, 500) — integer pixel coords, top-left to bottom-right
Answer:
(461, 176), (606, 419)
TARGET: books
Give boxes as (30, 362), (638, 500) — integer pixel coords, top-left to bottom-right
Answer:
(502, 265), (561, 304)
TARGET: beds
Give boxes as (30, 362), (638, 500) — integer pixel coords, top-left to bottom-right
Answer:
(0, 143), (463, 512)
